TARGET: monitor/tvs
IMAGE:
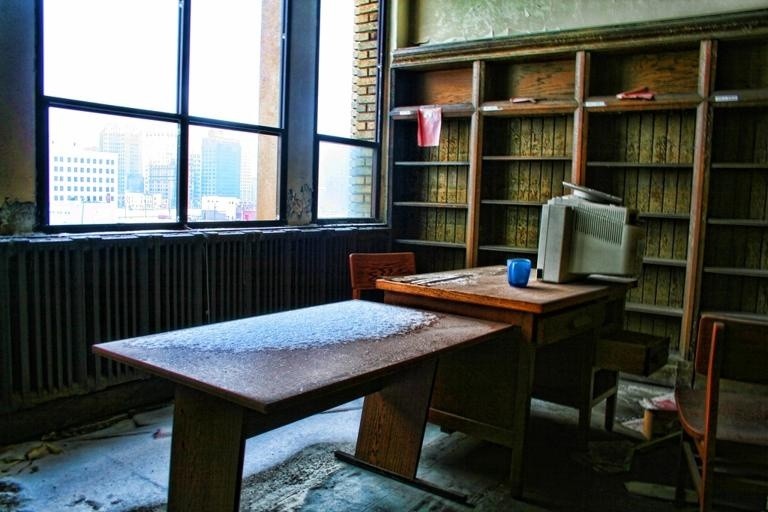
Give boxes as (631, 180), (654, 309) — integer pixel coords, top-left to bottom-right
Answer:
(536, 181), (641, 285)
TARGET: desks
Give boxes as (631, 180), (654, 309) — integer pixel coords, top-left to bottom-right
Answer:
(368, 258), (637, 502)
(92, 294), (526, 512)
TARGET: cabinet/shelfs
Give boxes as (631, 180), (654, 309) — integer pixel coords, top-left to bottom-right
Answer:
(382, 6), (765, 395)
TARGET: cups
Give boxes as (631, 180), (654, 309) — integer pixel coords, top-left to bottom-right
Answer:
(506, 258), (532, 287)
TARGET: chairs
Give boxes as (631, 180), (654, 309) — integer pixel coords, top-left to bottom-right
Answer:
(341, 243), (417, 304)
(673, 313), (766, 512)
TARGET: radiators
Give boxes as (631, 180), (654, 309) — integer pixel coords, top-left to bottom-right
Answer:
(0, 220), (391, 416)
